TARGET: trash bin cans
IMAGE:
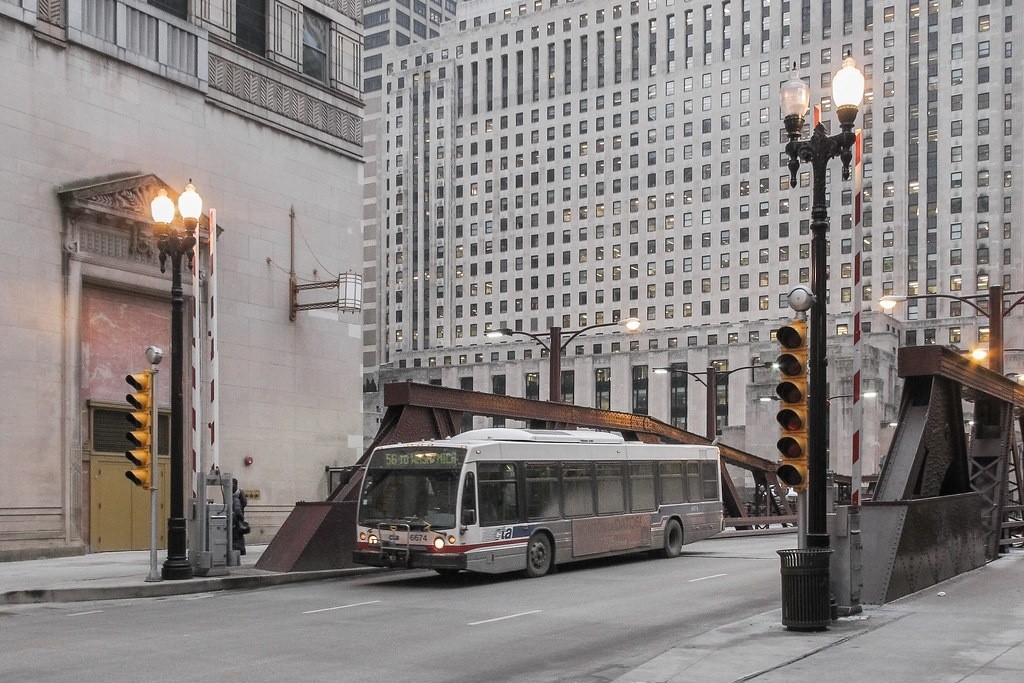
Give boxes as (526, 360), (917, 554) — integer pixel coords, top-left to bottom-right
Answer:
(776, 548), (836, 631)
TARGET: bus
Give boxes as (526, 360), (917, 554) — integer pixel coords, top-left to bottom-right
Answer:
(340, 426), (727, 580)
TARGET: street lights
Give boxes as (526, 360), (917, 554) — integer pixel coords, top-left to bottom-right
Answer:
(149, 175), (204, 579)
(488, 317), (640, 402)
(876, 284), (1024, 376)
(652, 361), (778, 441)
(777, 55), (867, 549)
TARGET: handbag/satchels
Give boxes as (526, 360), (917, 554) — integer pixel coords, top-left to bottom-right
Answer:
(235, 518), (250, 534)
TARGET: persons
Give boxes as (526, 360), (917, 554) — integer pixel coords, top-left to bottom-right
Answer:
(132, 222), (154, 256)
(232, 478), (247, 555)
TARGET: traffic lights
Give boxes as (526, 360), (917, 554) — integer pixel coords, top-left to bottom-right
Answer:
(776, 316), (808, 493)
(125, 370), (154, 491)
